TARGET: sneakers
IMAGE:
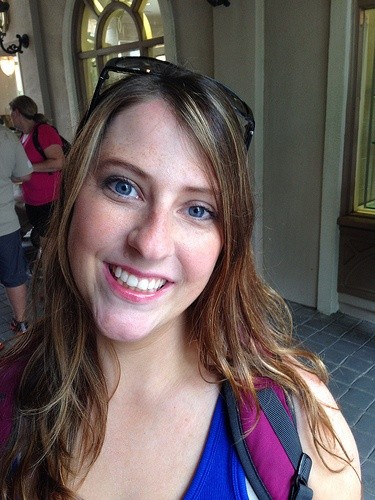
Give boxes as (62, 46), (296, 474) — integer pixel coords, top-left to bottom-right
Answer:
(9, 318), (27, 332)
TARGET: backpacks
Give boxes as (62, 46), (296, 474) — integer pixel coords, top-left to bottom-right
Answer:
(32, 122), (72, 176)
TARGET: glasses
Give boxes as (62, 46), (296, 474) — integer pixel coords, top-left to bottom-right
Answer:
(76, 55), (255, 151)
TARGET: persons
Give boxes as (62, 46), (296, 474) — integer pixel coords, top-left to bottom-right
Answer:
(9, 95), (65, 299)
(0, 55), (362, 500)
(0, 126), (34, 351)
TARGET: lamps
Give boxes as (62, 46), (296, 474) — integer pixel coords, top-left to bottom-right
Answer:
(0, 0), (29, 55)
